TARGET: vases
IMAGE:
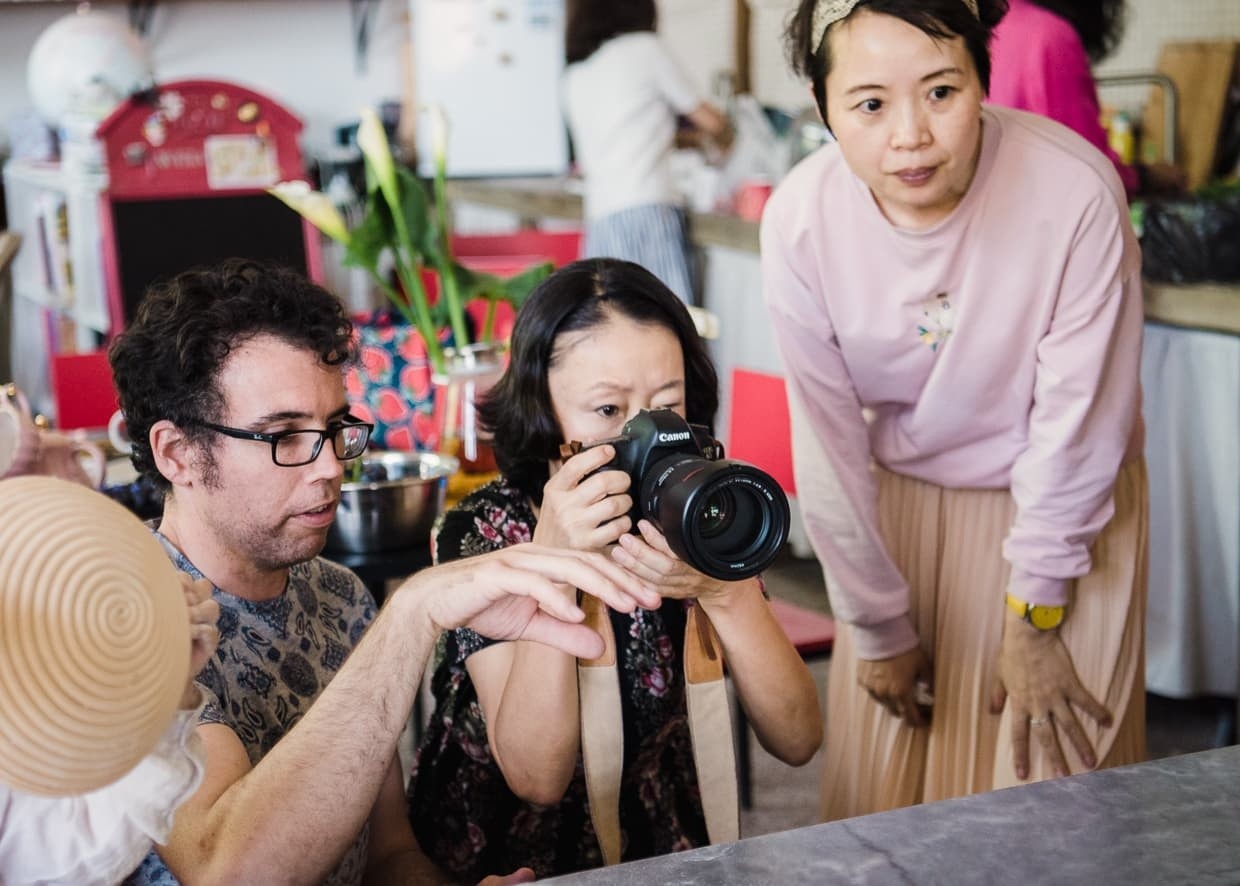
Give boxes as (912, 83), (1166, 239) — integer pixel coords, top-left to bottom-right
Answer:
(433, 341), (516, 468)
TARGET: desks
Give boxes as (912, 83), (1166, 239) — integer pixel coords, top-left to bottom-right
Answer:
(512, 744), (1240, 886)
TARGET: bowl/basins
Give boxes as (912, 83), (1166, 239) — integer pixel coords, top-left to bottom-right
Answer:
(331, 448), (460, 551)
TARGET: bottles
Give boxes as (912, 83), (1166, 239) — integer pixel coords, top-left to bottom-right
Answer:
(1108, 111), (1135, 163)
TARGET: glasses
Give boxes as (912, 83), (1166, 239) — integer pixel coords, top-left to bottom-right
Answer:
(171, 412), (376, 467)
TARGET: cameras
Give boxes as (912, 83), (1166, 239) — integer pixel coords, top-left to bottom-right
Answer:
(560, 405), (792, 582)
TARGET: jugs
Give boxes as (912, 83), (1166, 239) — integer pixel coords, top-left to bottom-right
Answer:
(34, 426), (109, 493)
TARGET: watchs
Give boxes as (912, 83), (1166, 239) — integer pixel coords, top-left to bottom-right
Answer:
(1003, 593), (1067, 632)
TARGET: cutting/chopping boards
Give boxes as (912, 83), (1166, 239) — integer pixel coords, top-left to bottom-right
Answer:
(1135, 35), (1239, 187)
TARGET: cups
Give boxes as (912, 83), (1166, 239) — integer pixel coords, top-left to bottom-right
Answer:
(107, 411), (139, 459)
(1, 381), (43, 481)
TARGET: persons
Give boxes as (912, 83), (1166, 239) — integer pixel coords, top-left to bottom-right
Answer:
(759, 0), (1149, 815)
(423, 255), (824, 880)
(0, 217), (666, 886)
(560, 0), (733, 305)
(989, 0), (1138, 193)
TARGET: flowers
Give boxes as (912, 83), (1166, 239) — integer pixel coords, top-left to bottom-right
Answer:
(276, 112), (519, 353)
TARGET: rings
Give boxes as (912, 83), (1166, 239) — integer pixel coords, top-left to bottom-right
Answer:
(1031, 718), (1046, 725)
